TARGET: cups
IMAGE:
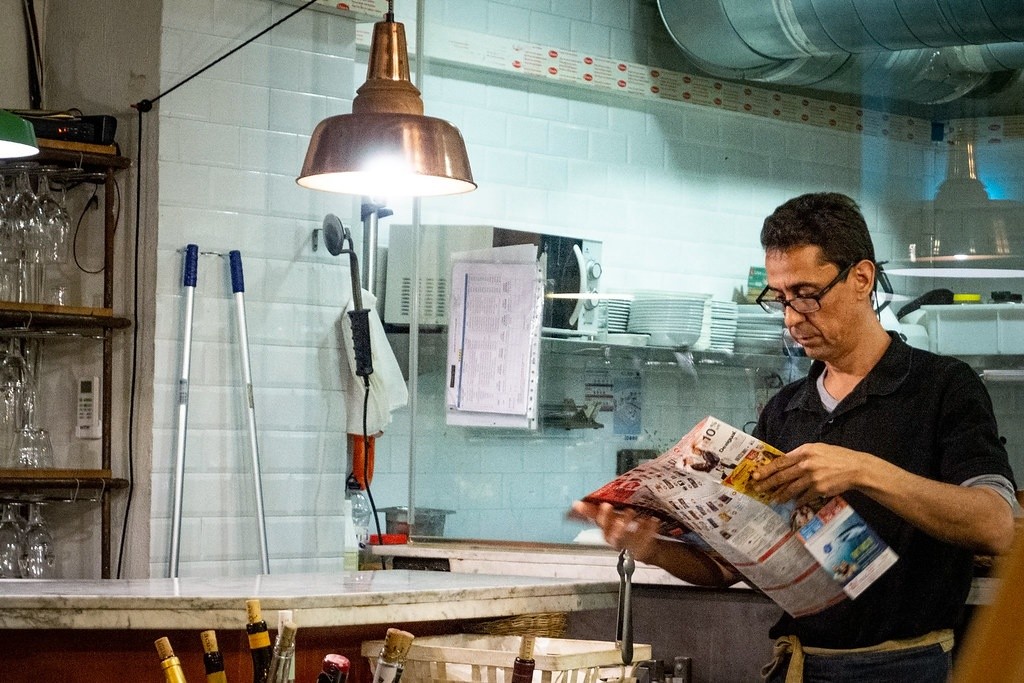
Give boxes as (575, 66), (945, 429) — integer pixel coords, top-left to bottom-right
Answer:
(7, 427), (53, 469)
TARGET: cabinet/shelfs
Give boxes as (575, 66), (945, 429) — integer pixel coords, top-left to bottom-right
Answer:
(0, 146), (134, 578)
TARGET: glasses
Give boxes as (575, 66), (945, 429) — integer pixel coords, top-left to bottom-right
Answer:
(755, 261), (856, 316)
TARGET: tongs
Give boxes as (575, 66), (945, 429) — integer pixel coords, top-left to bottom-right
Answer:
(615, 549), (635, 666)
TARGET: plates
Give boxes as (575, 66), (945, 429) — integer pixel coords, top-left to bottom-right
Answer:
(598, 290), (783, 354)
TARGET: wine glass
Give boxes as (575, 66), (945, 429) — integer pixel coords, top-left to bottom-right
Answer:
(0, 326), (41, 470)
(0, 493), (56, 579)
(0, 159), (73, 304)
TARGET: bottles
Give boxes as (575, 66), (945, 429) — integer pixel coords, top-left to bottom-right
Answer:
(154, 637), (186, 683)
(246, 600), (273, 683)
(345, 482), (370, 550)
(267, 624), (295, 683)
(201, 630), (227, 683)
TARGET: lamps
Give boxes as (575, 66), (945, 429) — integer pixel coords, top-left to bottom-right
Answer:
(910, 97), (1010, 260)
(0, 107), (39, 159)
(295, 0), (479, 197)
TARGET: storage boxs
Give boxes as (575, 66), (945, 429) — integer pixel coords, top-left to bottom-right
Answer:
(920, 303), (1022, 354)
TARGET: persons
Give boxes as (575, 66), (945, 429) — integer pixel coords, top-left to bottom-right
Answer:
(570, 191), (1021, 683)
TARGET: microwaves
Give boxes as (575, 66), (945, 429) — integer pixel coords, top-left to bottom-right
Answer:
(384, 224), (602, 335)
